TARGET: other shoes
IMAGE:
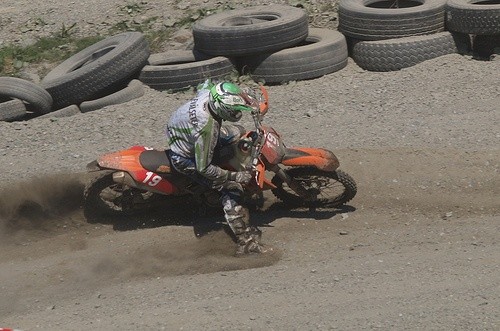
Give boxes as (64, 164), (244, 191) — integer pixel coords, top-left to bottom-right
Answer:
(233, 237), (276, 258)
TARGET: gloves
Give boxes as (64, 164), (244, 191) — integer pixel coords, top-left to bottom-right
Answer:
(243, 83), (258, 98)
(235, 171), (252, 183)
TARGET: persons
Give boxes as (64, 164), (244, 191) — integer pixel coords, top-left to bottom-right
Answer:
(166, 79), (273, 257)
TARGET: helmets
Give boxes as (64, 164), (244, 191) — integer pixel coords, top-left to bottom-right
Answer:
(208, 81), (256, 121)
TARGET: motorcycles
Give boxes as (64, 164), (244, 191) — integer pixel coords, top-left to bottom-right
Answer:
(81, 82), (357, 215)
